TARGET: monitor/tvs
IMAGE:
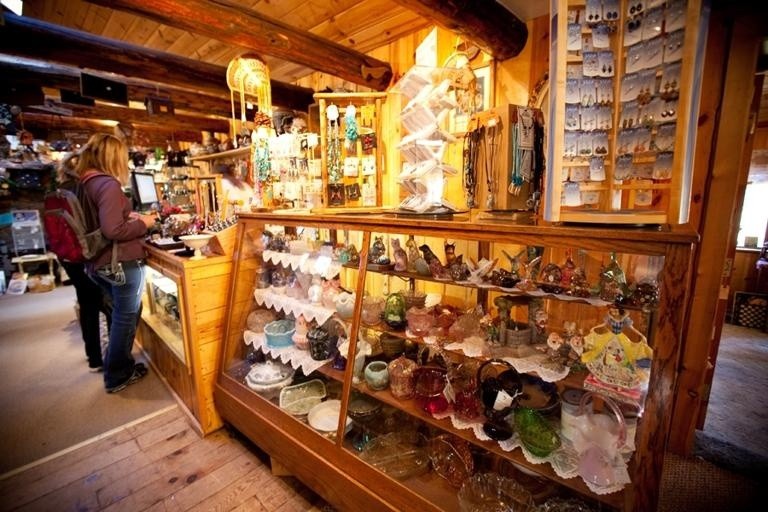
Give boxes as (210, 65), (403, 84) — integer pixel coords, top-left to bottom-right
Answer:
(131, 170), (158, 206)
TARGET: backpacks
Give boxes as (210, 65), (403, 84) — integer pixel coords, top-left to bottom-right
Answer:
(39, 172), (114, 264)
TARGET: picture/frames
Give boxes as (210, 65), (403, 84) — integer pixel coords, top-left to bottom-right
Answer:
(445, 59), (496, 134)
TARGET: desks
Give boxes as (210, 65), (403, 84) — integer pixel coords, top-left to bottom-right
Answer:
(11, 253), (56, 289)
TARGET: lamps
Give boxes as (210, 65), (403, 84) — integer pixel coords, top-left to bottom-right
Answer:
(222, 51), (275, 149)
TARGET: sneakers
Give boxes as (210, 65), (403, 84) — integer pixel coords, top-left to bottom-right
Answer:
(88, 359), (149, 393)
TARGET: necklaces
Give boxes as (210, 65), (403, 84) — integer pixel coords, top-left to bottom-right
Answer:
(326, 118), (342, 184)
(461, 113), (544, 214)
(251, 138), (272, 203)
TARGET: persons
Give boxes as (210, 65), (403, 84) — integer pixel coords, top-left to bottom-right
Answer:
(57, 152), (114, 374)
(75, 133), (160, 393)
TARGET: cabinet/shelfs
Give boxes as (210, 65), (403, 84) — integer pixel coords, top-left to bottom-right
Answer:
(213, 210), (700, 512)
